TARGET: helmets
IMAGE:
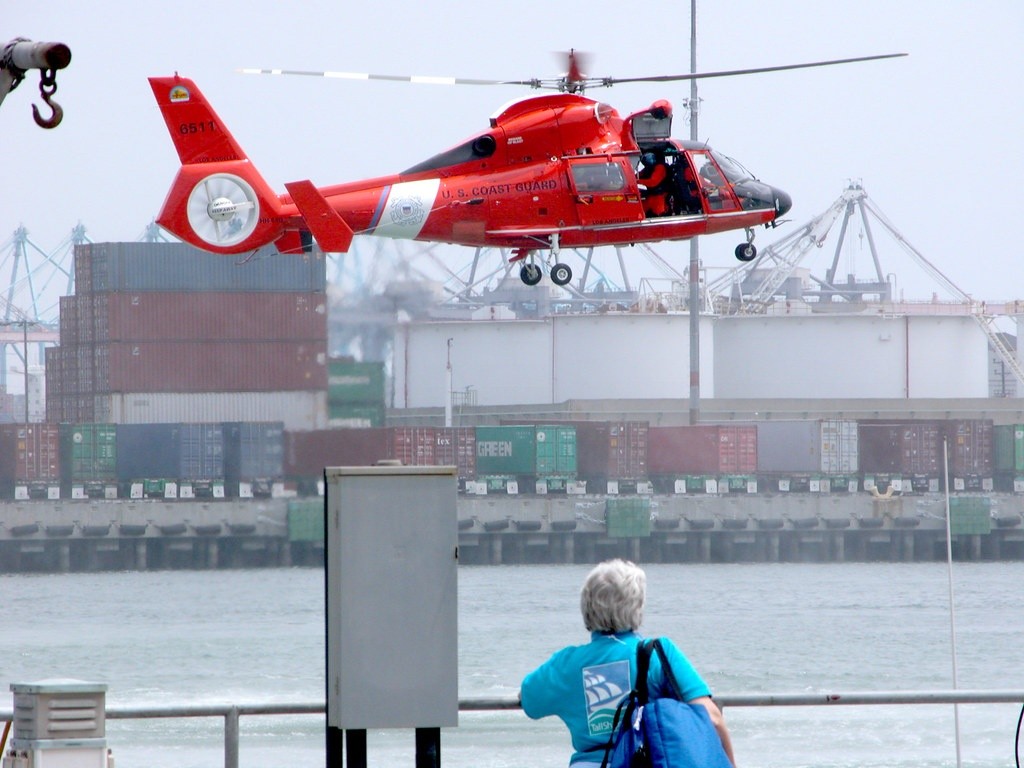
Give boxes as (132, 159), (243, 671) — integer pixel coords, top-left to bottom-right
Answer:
(640, 150), (655, 165)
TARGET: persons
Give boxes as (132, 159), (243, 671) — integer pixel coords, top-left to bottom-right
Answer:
(635, 151), (715, 218)
(518, 559), (736, 768)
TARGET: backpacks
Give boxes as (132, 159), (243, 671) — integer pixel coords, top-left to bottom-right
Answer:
(599, 640), (731, 768)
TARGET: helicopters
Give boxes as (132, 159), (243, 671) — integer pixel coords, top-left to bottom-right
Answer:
(148, 46), (909, 288)
